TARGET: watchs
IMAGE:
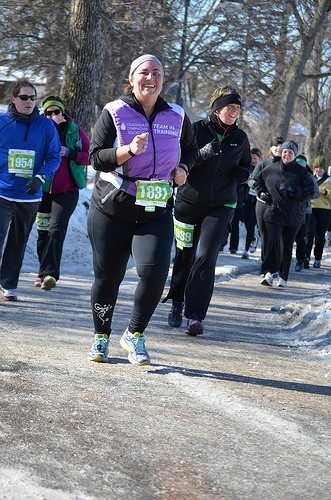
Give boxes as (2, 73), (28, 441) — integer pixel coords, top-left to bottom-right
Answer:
(126, 145), (136, 157)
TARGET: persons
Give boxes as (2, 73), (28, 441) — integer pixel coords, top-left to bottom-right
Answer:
(87, 54), (199, 366)
(0, 74), (331, 336)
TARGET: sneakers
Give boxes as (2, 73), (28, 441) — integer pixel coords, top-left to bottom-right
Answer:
(35, 276), (56, 290)
(277, 276), (287, 287)
(168, 300), (184, 328)
(0, 283), (17, 301)
(89, 333), (110, 363)
(260, 272), (274, 285)
(185, 318), (204, 337)
(120, 327), (151, 365)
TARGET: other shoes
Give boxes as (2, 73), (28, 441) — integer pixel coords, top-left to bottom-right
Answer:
(295, 262), (304, 272)
(303, 258), (310, 269)
(313, 260), (321, 268)
(249, 235), (259, 253)
(242, 251), (248, 259)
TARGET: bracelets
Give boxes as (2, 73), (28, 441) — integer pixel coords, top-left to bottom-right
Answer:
(179, 165), (188, 176)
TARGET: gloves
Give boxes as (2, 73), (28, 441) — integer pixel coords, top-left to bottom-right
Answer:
(26, 177), (43, 195)
(234, 168), (250, 183)
(199, 139), (223, 161)
(260, 190), (271, 202)
(280, 183), (294, 194)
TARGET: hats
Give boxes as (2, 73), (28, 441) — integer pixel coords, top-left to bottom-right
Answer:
(296, 155), (307, 168)
(281, 140), (298, 156)
(250, 148), (261, 158)
(313, 155), (326, 170)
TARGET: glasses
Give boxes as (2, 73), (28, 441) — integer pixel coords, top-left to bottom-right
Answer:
(17, 95), (37, 101)
(45, 109), (62, 116)
